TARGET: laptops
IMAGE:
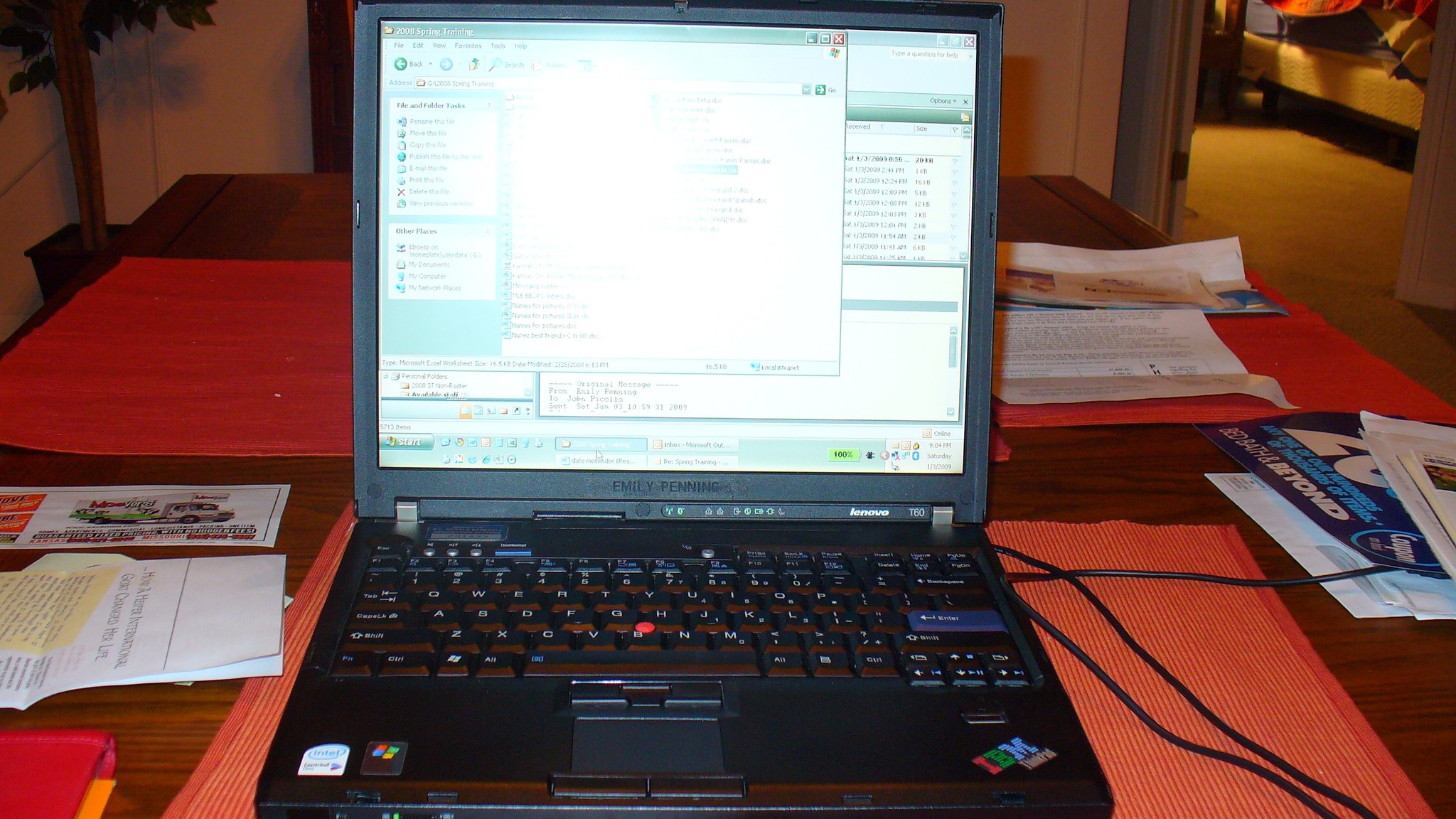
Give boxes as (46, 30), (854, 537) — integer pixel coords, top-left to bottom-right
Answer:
(255, 0), (1113, 819)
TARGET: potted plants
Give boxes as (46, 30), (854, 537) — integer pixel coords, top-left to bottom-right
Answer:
(0, 1), (215, 305)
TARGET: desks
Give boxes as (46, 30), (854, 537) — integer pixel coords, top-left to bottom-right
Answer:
(0, 173), (1456, 819)
